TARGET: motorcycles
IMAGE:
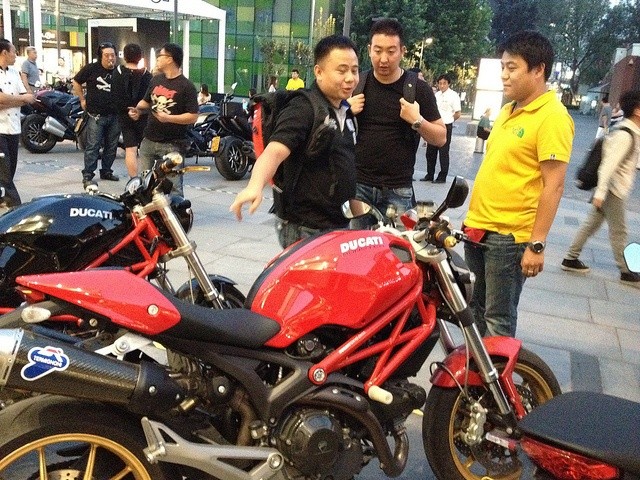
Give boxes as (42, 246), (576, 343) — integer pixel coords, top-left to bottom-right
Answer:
(67, 96), (140, 159)
(1, 175), (562, 480)
(206, 94), (259, 180)
(184, 82), (239, 164)
(19, 90), (87, 153)
(1, 151), (246, 414)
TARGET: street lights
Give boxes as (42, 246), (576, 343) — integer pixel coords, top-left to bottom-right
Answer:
(419, 36), (433, 69)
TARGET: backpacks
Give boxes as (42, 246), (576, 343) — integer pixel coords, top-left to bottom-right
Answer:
(253, 88), (359, 192)
(574, 126), (634, 188)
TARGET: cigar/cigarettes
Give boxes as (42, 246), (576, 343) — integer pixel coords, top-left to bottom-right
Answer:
(35, 99), (42, 102)
(110, 62), (113, 65)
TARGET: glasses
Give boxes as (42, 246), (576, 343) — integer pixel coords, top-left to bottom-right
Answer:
(156, 53), (171, 59)
(99, 42), (114, 50)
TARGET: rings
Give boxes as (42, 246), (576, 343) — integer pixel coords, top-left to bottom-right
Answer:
(527, 270), (534, 273)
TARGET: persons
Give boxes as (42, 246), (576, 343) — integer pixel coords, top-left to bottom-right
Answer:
(561, 89), (640, 282)
(589, 95), (600, 116)
(111, 42), (154, 177)
(408, 68), (425, 83)
(347, 19), (447, 230)
(197, 84), (211, 105)
(477, 106), (493, 140)
(0, 39), (36, 209)
(610, 102), (623, 126)
(594, 96), (612, 139)
(70, 42), (120, 183)
(126, 43), (199, 198)
(242, 88), (258, 113)
(53, 56), (70, 84)
(576, 93), (582, 107)
(269, 77), (278, 95)
(462, 28), (575, 342)
(21, 46), (40, 92)
(228, 35), (360, 254)
(286, 69), (304, 91)
(418, 73), (462, 184)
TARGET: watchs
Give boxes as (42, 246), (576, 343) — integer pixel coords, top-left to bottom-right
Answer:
(410, 116), (424, 130)
(527, 240), (547, 253)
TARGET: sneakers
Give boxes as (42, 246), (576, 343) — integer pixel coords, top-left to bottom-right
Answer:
(618, 271), (640, 290)
(560, 258), (592, 274)
